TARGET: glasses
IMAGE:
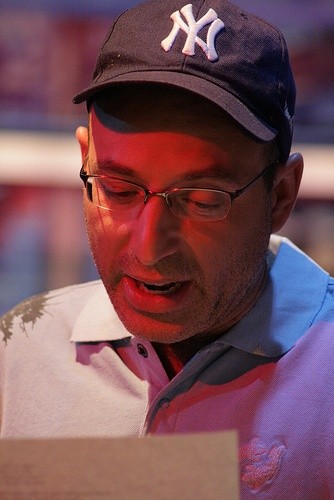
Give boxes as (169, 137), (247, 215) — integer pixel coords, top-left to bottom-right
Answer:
(78, 154), (277, 221)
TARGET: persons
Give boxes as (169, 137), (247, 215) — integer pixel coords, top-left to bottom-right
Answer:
(1, 0), (334, 500)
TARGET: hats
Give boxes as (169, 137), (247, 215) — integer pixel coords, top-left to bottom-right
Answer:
(73, 0), (299, 144)
